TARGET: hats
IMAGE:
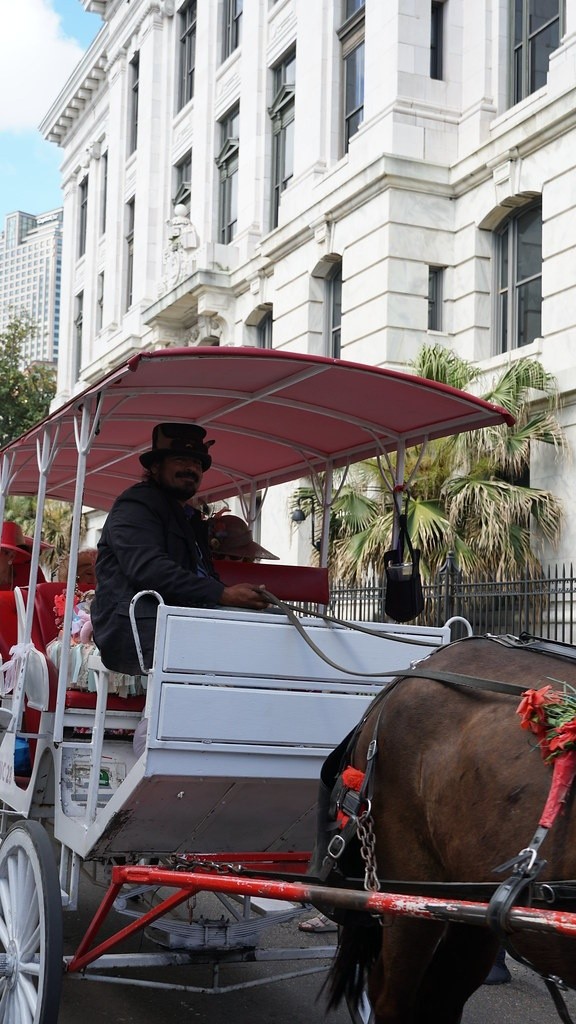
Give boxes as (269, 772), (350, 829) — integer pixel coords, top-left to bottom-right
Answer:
(0, 521), (31, 563)
(139, 423), (216, 473)
(23, 536), (55, 550)
(58, 549), (97, 583)
(207, 508), (280, 560)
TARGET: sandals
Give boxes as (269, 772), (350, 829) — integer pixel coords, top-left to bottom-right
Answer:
(299, 913), (339, 933)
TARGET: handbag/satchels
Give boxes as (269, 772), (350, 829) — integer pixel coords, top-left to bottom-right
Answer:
(383, 486), (424, 623)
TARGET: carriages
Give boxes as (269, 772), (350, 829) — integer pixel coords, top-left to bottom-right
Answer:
(1, 339), (576, 1024)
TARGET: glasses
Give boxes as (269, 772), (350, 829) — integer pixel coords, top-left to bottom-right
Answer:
(215, 554), (243, 560)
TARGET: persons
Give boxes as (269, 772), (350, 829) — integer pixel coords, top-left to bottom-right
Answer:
(206, 515), (279, 563)
(298, 912), (338, 932)
(89, 423), (269, 680)
(57, 548), (98, 584)
(0, 522), (31, 591)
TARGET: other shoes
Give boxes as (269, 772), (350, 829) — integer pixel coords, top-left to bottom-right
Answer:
(484, 952), (511, 984)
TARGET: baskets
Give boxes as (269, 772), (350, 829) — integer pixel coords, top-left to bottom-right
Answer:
(46, 590), (146, 699)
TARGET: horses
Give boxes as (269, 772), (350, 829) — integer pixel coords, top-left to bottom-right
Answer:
(317, 636), (576, 1024)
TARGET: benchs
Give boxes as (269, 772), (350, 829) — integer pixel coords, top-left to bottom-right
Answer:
(0, 559), (359, 740)
